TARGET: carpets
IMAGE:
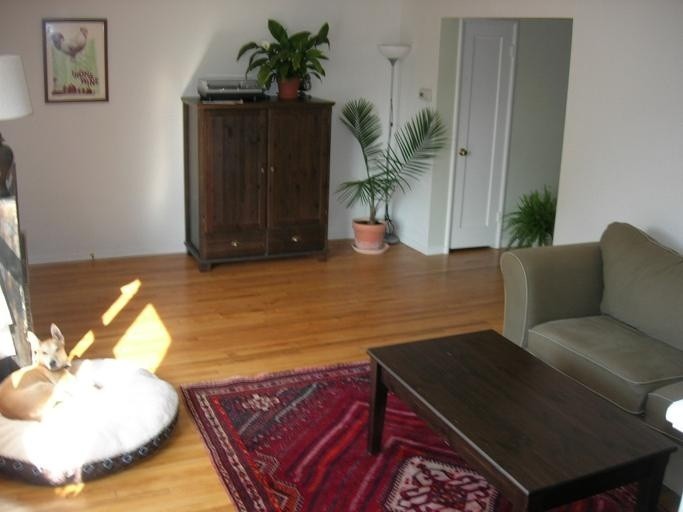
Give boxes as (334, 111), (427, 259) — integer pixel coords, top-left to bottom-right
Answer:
(180, 362), (667, 512)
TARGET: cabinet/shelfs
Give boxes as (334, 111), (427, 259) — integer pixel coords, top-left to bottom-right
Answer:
(181, 94), (336, 273)
(452, 19), (518, 254)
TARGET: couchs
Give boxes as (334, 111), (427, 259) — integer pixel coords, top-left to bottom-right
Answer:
(499, 221), (683, 496)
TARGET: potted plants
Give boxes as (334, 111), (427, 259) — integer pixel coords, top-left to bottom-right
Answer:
(332, 97), (451, 255)
(236, 19), (331, 100)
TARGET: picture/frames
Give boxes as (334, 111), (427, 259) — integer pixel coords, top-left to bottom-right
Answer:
(41, 18), (108, 103)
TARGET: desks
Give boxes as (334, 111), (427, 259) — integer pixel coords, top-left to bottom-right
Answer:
(365, 329), (676, 511)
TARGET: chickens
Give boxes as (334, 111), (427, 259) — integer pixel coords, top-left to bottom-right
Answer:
(51, 26), (89, 61)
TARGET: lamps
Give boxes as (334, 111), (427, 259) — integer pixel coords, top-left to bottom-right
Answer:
(0, 55), (35, 200)
(378, 45), (410, 245)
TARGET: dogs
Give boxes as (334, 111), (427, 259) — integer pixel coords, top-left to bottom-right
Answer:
(0, 322), (71, 421)
